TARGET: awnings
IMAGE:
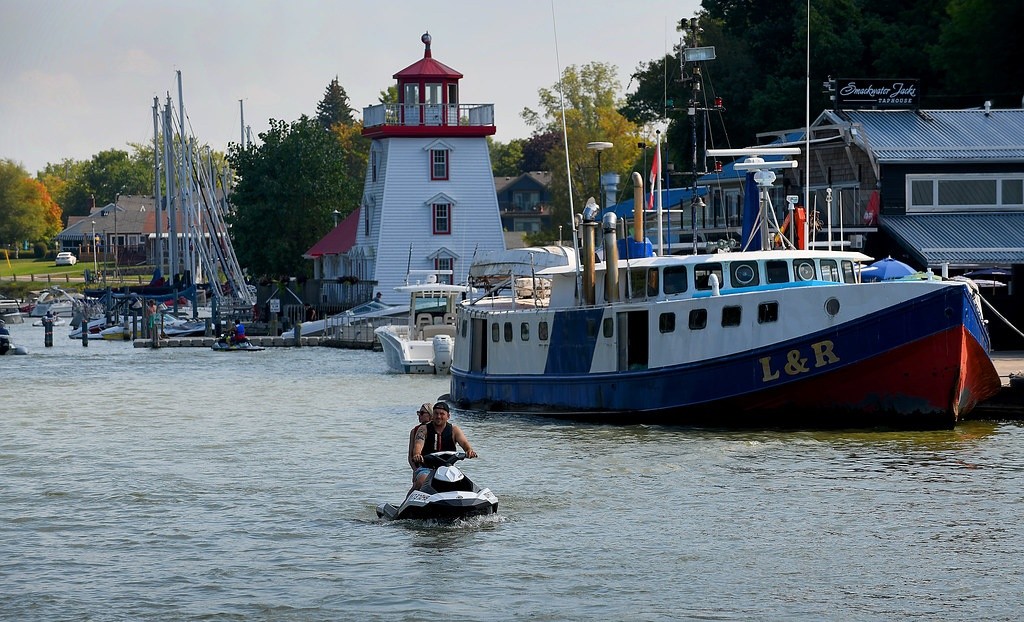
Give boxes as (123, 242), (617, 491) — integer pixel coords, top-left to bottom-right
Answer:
(877, 214), (1024, 267)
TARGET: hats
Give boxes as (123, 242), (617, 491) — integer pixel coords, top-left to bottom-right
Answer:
(422, 403), (434, 417)
(433, 402), (449, 414)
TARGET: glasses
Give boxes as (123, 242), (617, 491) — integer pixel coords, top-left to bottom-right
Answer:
(417, 411), (429, 416)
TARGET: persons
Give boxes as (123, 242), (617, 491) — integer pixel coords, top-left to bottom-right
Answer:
(409, 402), (476, 486)
(373, 292), (382, 302)
(0, 320), (9, 335)
(15, 243), (19, 258)
(46, 311), (56, 318)
(307, 304), (316, 322)
(223, 319), (246, 346)
(146, 299), (163, 340)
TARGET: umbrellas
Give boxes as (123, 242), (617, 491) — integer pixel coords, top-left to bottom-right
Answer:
(962, 266), (1015, 296)
(854, 255), (918, 281)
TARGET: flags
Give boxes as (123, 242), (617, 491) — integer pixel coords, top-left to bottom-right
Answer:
(648, 130), (660, 209)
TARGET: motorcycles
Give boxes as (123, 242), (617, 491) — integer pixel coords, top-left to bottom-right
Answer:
(211, 334), (265, 351)
(377, 451), (499, 520)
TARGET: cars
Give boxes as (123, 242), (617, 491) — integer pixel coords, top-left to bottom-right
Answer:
(55, 252), (77, 266)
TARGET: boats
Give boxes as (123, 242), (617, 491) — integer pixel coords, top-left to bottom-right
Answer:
(449, 0), (1002, 428)
(282, 298), (448, 341)
(68, 307), (215, 341)
(0, 334), (28, 355)
(32, 316), (65, 327)
(373, 205), (479, 374)
(31, 286), (78, 317)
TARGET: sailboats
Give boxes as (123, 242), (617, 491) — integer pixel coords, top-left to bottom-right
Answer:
(83, 70), (258, 317)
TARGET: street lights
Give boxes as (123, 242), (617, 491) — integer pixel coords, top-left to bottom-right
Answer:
(92, 221), (98, 270)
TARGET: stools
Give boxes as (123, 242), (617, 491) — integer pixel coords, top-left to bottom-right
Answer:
(416, 313), (432, 331)
(444, 313), (457, 326)
